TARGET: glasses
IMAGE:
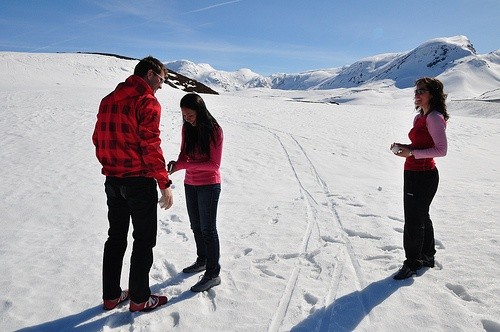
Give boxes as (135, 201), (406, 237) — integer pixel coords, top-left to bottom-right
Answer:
(153, 71), (165, 84)
(415, 88), (428, 94)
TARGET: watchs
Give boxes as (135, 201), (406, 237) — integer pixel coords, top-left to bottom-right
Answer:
(409, 151), (413, 157)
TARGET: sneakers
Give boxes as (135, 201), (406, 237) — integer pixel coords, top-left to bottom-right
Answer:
(182, 262), (207, 273)
(190, 272), (221, 292)
(129, 295), (167, 312)
(102, 290), (129, 310)
(420, 257), (434, 268)
(393, 261), (423, 280)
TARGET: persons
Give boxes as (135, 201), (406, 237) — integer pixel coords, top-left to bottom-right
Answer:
(91, 56), (173, 313)
(390, 77), (450, 280)
(169, 93), (224, 293)
